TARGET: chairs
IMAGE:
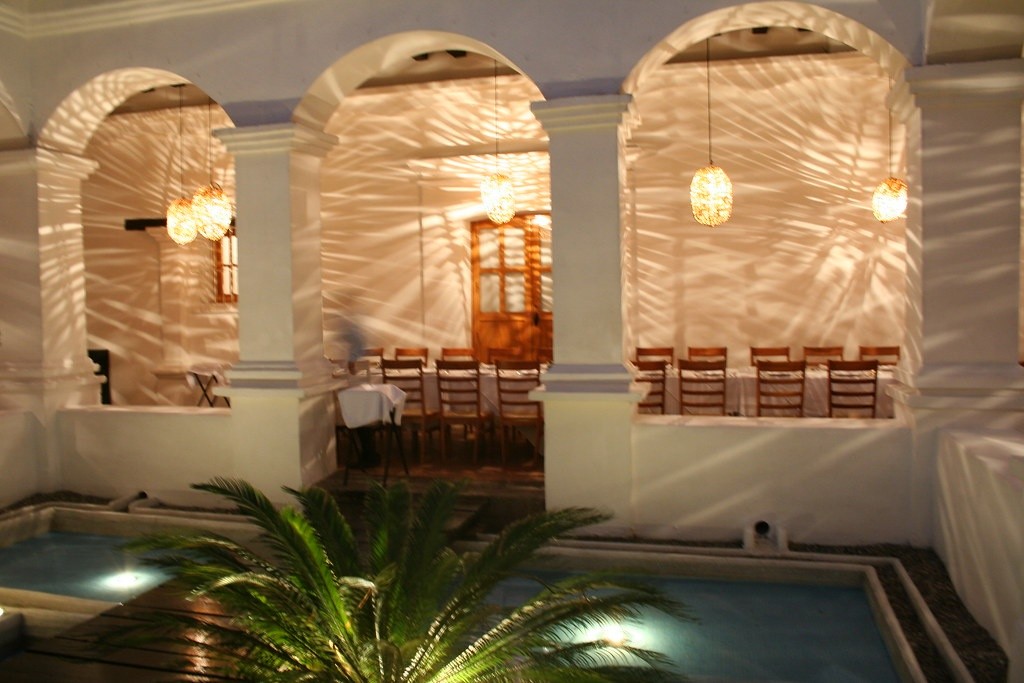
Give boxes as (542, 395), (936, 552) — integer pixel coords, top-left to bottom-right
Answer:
(688, 347), (729, 368)
(394, 347), (428, 368)
(860, 346), (901, 366)
(487, 347), (524, 369)
(755, 360), (808, 418)
(328, 347), (383, 379)
(631, 361), (666, 415)
(496, 359), (545, 462)
(435, 359), (494, 462)
(441, 347), (478, 368)
(676, 358), (727, 417)
(634, 346), (675, 368)
(827, 359), (878, 421)
(751, 347), (791, 366)
(379, 358), (441, 460)
(802, 347), (843, 363)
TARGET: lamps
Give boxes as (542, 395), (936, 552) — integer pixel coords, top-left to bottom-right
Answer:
(166, 82), (199, 244)
(689, 37), (732, 228)
(871, 74), (908, 224)
(190, 91), (233, 242)
(479, 60), (516, 225)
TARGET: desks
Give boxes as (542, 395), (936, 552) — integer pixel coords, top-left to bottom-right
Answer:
(337, 383), (412, 488)
(638, 366), (893, 419)
(188, 361), (231, 408)
(335, 368), (545, 461)
